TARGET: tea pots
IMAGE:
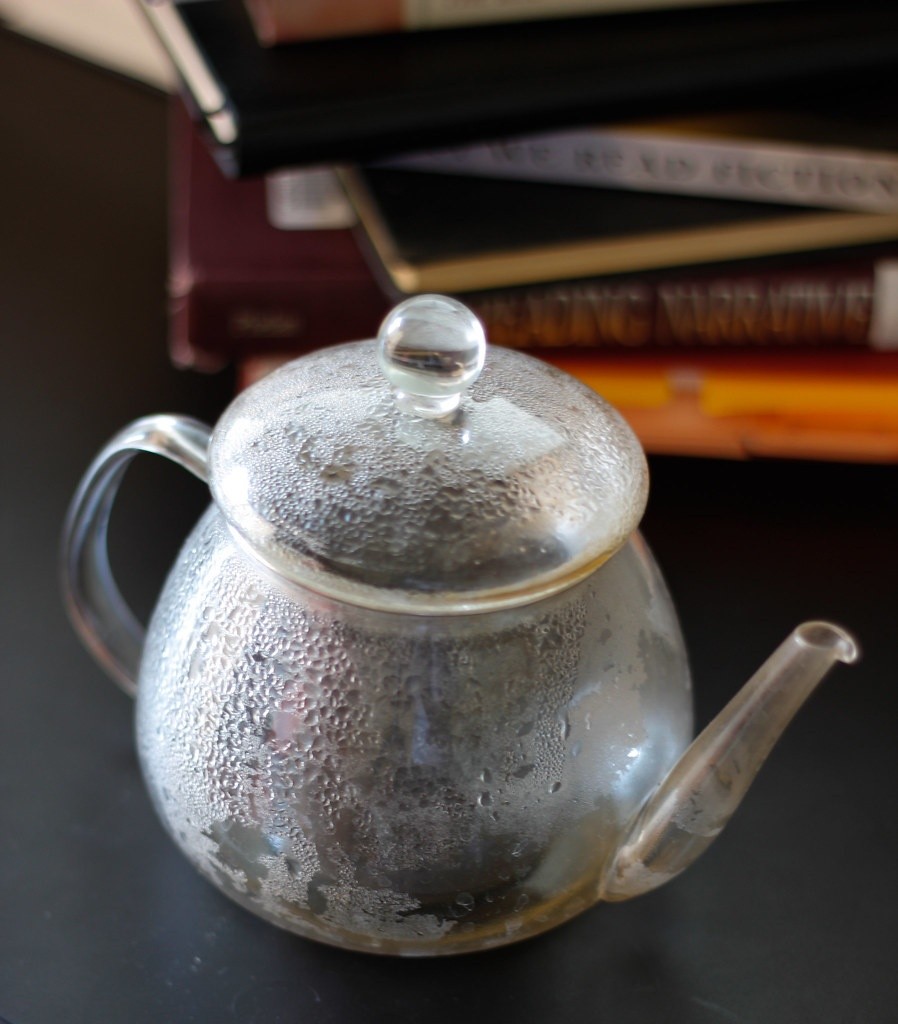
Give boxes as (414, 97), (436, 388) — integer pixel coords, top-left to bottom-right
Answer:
(59, 293), (862, 958)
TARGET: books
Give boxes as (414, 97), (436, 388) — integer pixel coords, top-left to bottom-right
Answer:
(130, 1), (898, 464)
